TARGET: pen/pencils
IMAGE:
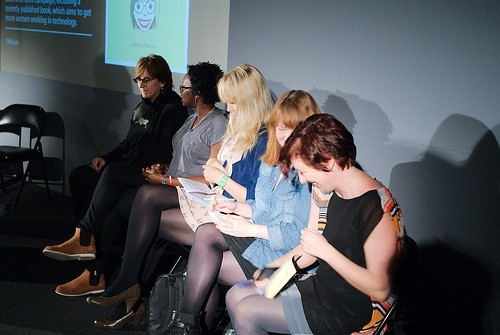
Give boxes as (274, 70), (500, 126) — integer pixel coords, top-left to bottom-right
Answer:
(223, 160), (228, 168)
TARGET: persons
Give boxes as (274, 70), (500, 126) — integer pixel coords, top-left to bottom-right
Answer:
(179, 89), (321, 315)
(225, 113), (406, 335)
(43, 61), (230, 275)
(69, 54), (189, 296)
(86, 64), (276, 328)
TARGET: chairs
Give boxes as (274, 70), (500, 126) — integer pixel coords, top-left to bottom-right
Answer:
(0, 104), (66, 214)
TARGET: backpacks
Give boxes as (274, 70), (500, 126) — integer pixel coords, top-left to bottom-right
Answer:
(145, 268), (202, 335)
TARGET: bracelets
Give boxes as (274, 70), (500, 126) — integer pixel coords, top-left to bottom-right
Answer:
(162, 176), (166, 184)
(169, 177), (172, 184)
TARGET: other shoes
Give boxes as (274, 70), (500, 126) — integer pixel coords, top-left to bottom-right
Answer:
(43, 226), (96, 260)
(55, 267), (105, 296)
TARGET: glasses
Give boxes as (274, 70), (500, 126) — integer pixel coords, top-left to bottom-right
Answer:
(180, 86), (193, 92)
(133, 75), (157, 83)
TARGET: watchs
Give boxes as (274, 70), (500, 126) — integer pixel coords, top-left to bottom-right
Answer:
(218, 175), (227, 187)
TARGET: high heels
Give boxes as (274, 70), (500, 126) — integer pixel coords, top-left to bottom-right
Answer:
(85, 284), (140, 313)
(94, 303), (145, 329)
